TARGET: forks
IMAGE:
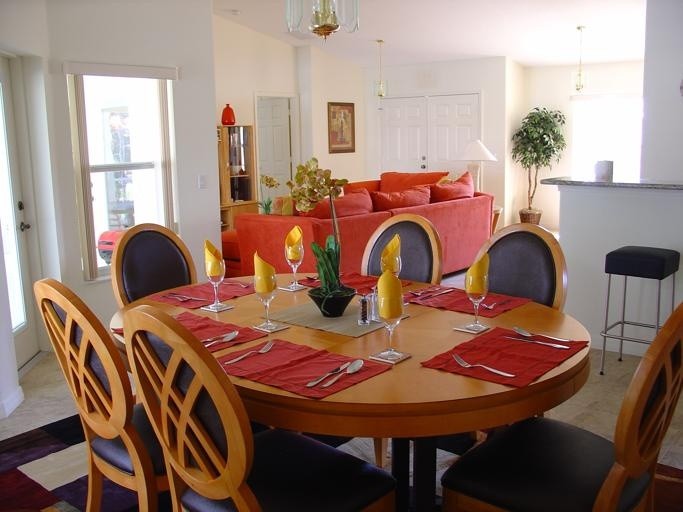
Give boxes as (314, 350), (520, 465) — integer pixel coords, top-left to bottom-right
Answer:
(480, 299), (512, 310)
(452, 353), (516, 377)
(224, 340), (275, 366)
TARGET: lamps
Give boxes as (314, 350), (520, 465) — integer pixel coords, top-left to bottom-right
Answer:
(286, 0), (357, 42)
(372, 31), (389, 100)
(454, 140), (496, 191)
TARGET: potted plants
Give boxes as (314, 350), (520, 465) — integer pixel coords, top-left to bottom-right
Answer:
(512, 106), (567, 225)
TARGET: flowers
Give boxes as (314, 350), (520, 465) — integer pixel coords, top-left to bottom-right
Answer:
(285, 156), (350, 294)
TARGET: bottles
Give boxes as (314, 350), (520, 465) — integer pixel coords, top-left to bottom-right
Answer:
(221, 103), (235, 125)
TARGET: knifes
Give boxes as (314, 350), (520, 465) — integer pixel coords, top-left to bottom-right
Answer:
(199, 332), (232, 342)
(504, 336), (570, 350)
(304, 362), (350, 389)
(169, 292), (209, 301)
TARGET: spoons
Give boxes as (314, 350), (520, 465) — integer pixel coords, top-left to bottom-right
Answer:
(205, 330), (239, 348)
(319, 359), (363, 389)
(512, 326), (571, 343)
(159, 295), (192, 302)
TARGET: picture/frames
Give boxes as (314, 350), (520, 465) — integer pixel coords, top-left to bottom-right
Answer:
(327, 101), (355, 154)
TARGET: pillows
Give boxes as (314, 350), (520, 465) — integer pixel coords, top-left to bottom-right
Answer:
(290, 173), (477, 221)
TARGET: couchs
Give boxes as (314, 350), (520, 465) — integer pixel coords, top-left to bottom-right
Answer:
(222, 171), (493, 277)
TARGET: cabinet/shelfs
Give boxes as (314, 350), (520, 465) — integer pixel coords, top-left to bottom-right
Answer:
(217, 125), (257, 233)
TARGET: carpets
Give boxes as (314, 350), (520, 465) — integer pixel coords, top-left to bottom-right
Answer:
(0, 412), (666, 512)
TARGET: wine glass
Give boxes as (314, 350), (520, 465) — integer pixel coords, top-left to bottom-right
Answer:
(204, 243), (489, 361)
